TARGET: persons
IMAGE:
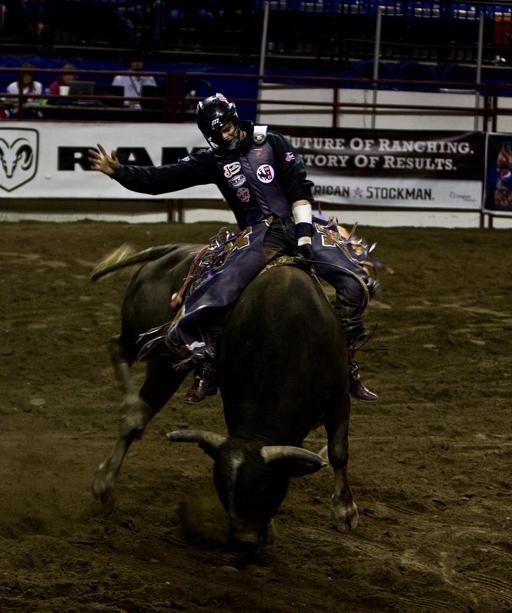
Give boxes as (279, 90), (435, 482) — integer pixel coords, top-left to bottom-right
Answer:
(112, 62), (157, 109)
(494, 142), (512, 208)
(48, 63), (78, 106)
(88, 93), (378, 402)
(4, 62), (43, 118)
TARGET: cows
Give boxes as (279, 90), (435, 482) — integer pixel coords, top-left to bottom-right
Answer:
(84, 241), (362, 568)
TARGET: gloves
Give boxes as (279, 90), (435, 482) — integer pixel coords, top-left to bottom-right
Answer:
(295, 244), (315, 271)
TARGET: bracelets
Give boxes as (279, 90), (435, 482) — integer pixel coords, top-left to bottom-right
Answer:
(295, 222), (314, 239)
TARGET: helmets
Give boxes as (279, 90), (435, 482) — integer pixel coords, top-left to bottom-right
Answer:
(195, 94), (239, 142)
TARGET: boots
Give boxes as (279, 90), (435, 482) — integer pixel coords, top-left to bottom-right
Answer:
(348, 343), (379, 403)
(184, 358), (217, 404)
